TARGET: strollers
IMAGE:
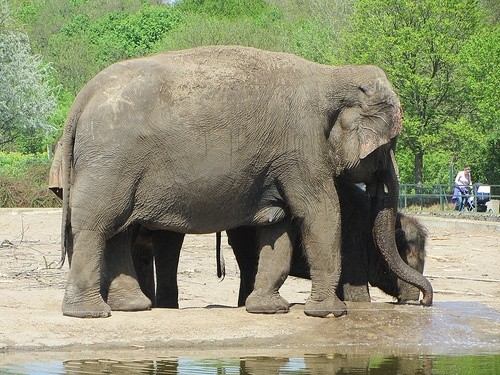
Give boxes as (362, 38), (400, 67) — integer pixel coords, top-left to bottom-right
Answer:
(460, 183), (493, 213)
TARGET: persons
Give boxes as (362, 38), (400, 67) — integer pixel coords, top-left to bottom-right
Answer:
(454, 165), (472, 212)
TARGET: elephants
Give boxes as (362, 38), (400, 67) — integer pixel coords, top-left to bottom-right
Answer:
(215, 183), (426, 306)
(48, 135), (186, 312)
(56, 44), (434, 317)
